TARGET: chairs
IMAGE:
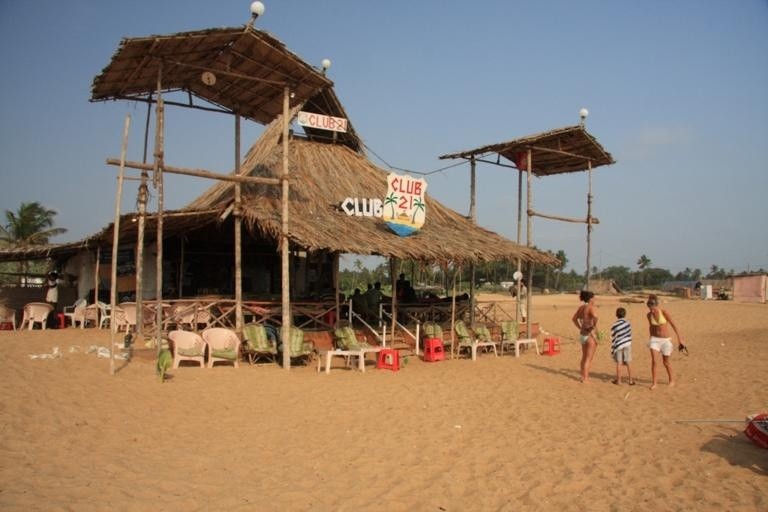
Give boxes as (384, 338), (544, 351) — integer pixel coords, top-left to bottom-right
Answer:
(167, 302), (562, 376)
(2, 300), (168, 335)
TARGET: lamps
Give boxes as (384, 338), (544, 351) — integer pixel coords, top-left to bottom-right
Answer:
(248, 1), (265, 26)
(580, 108), (588, 127)
(290, 88), (295, 98)
(322, 59), (331, 73)
(131, 203), (138, 222)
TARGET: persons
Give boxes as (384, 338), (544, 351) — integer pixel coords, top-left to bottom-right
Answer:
(519, 279), (527, 323)
(609, 307), (636, 385)
(645, 294), (686, 391)
(571, 289), (599, 383)
(44, 270), (60, 328)
(338, 272), (469, 325)
